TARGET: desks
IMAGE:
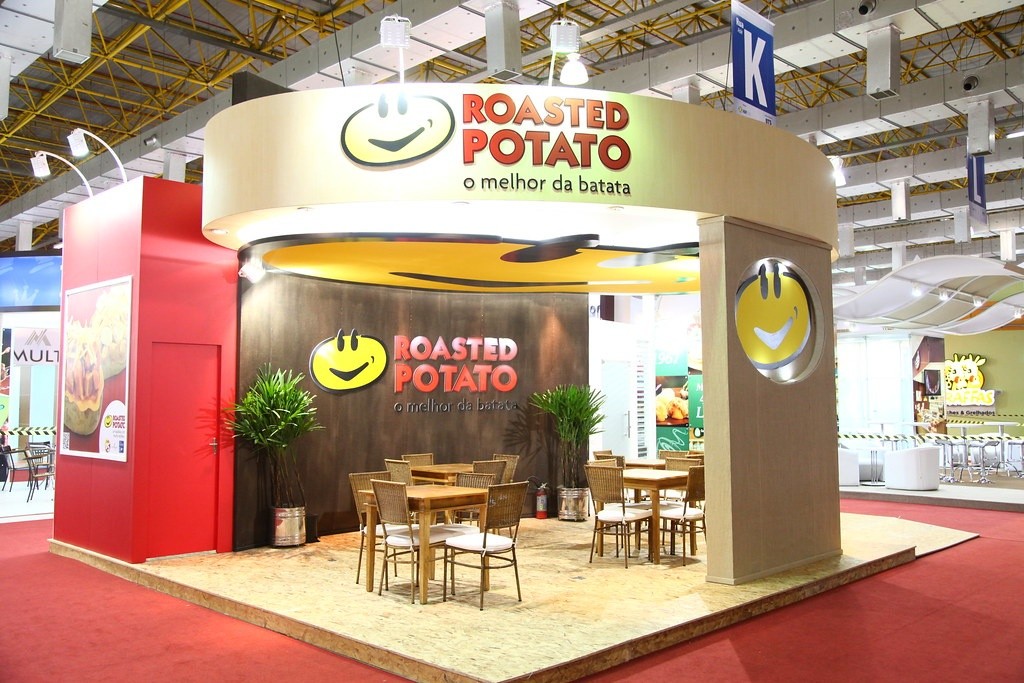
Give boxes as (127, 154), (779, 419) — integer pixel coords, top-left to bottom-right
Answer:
(597, 469), (696, 565)
(410, 464), (473, 525)
(357, 484), (489, 605)
(625, 459), (665, 470)
(861, 446), (886, 487)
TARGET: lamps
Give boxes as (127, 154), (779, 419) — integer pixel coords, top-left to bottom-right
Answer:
(560, 52), (589, 85)
(67, 128), (127, 183)
(30, 150), (93, 197)
(939, 287), (948, 300)
(380, 14), (412, 83)
(973, 295), (981, 307)
(830, 156), (846, 186)
(548, 17), (580, 86)
(1014, 306), (1022, 318)
(912, 281), (921, 297)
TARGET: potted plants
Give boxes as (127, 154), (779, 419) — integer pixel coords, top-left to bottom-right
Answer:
(221, 362), (326, 547)
(529, 384), (607, 521)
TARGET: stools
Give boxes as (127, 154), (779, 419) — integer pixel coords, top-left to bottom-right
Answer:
(935, 439), (1024, 484)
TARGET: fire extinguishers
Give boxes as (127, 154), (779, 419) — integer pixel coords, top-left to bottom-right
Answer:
(527, 475), (551, 519)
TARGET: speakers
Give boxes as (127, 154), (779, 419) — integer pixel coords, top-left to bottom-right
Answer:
(600, 295), (615, 321)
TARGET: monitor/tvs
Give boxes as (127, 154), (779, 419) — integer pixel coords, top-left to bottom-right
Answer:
(924, 370), (941, 396)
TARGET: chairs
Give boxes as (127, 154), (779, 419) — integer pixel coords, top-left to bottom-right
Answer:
(838, 448), (859, 486)
(584, 450), (706, 569)
(349, 453), (529, 611)
(0, 441), (55, 502)
(884, 446), (940, 491)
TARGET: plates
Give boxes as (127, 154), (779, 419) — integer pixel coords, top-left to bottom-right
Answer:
(657, 388), (689, 427)
